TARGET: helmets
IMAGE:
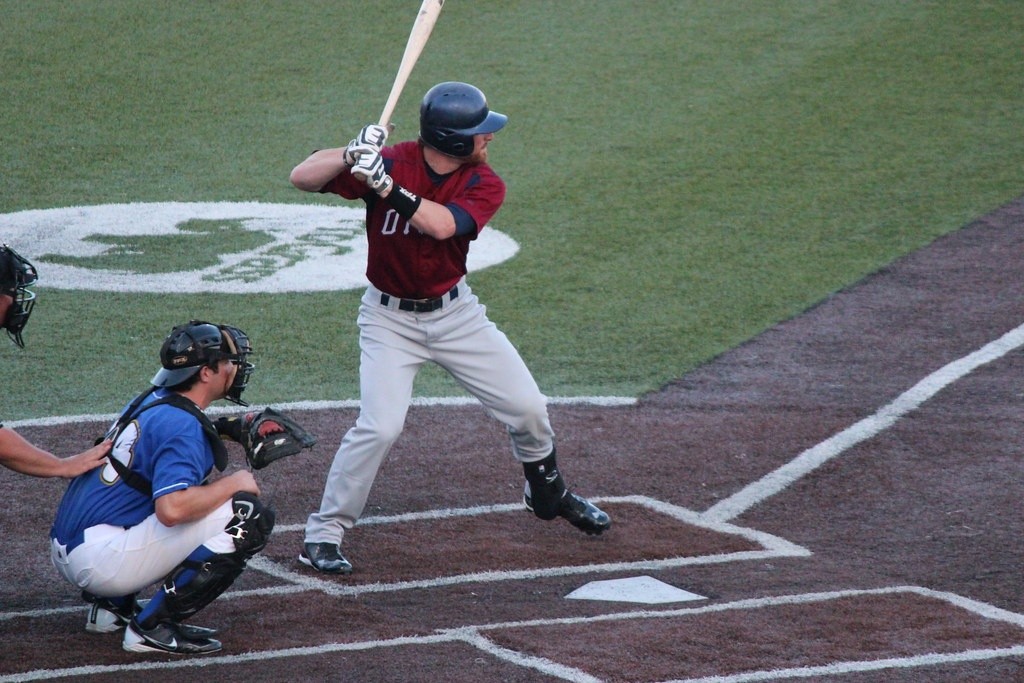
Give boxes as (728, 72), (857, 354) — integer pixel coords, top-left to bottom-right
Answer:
(0, 245), (35, 287)
(419, 81), (509, 157)
(149, 321), (222, 387)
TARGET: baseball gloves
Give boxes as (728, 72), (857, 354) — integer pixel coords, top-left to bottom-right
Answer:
(246, 407), (318, 469)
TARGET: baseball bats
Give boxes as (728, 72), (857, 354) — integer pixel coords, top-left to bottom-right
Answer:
(353, 0), (445, 181)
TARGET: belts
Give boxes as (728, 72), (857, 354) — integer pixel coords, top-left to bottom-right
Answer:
(381, 287), (458, 313)
(51, 531), (85, 555)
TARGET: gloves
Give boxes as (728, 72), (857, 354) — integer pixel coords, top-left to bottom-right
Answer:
(348, 146), (393, 193)
(348, 122), (395, 151)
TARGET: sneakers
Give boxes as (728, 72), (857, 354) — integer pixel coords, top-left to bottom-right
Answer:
(297, 542), (353, 574)
(122, 619), (221, 655)
(85, 606), (132, 634)
(523, 489), (610, 536)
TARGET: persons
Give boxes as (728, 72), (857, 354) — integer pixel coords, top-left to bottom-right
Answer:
(291, 82), (612, 575)
(51, 321), (316, 657)
(0, 244), (111, 477)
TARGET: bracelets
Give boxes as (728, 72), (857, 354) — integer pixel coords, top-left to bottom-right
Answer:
(342, 146), (354, 168)
